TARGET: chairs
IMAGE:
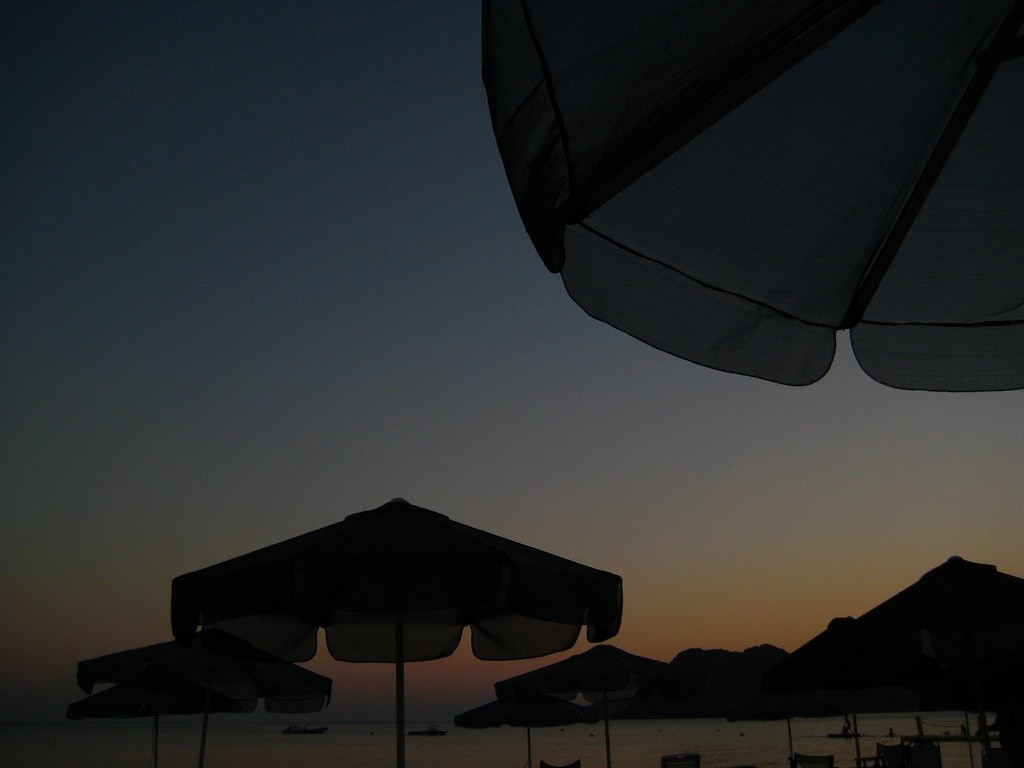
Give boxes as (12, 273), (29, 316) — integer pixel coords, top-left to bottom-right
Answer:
(788, 736), (941, 768)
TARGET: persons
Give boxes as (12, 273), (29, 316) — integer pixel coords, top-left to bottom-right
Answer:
(842, 726), (849, 734)
(960, 724), (967, 736)
(844, 717), (852, 734)
(889, 728), (896, 737)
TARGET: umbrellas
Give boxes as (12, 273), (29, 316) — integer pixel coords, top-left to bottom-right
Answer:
(761, 555), (1024, 768)
(479, 0), (1024, 395)
(614, 643), (842, 760)
(172, 498), (623, 768)
(76, 627), (333, 768)
(65, 684), (241, 768)
(455, 694), (601, 768)
(494, 644), (707, 768)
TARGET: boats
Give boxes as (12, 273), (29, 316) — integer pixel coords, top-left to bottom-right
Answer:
(408, 726), (449, 736)
(827, 727), (862, 739)
(281, 721), (329, 735)
